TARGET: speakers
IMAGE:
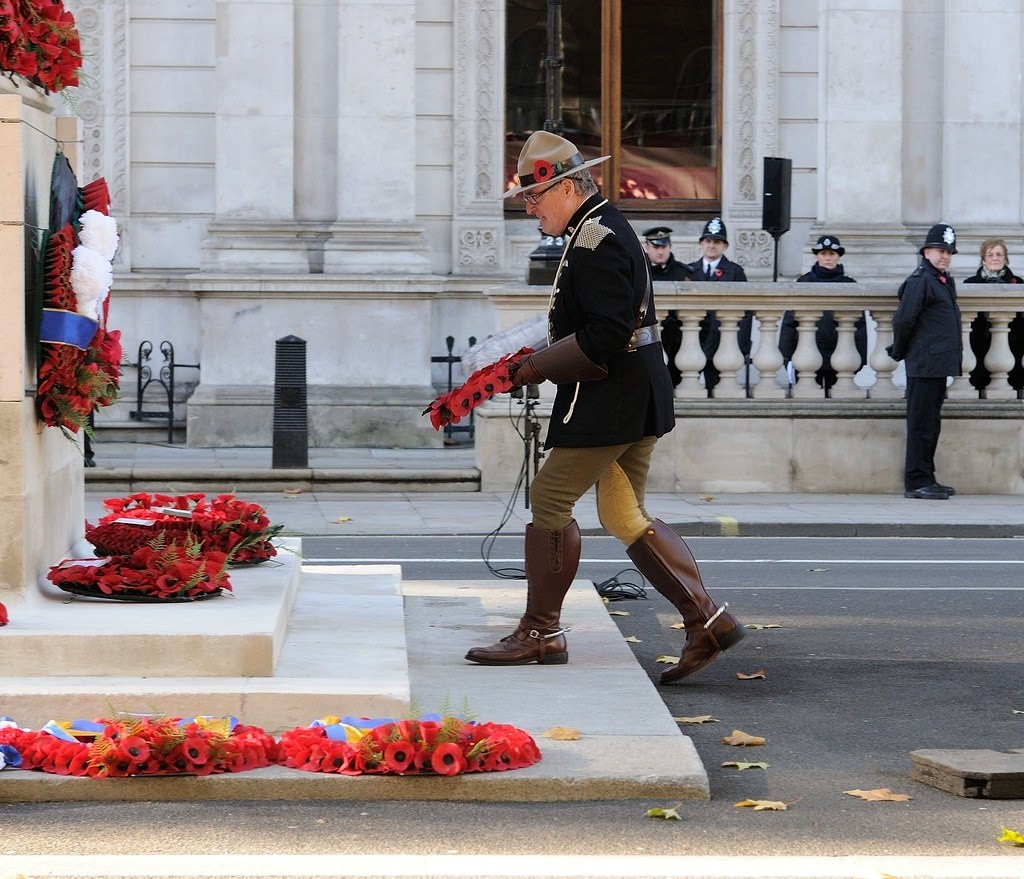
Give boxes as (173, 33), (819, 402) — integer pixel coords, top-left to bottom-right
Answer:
(761, 157), (792, 239)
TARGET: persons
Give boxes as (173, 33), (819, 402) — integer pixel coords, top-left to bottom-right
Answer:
(961, 239), (1024, 400)
(885, 224), (964, 500)
(641, 226), (703, 400)
(687, 216), (754, 400)
(778, 235), (869, 398)
(466, 131), (746, 684)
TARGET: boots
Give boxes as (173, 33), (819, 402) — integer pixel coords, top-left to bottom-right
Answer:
(465, 518), (581, 666)
(625, 518), (746, 685)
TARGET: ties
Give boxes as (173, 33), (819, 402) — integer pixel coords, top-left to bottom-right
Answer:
(704, 264), (710, 281)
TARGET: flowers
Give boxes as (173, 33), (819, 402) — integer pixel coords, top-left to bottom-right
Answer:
(1, 695), (541, 777)
(421, 344), (539, 432)
(43, 495), (302, 603)
(32, 177), (127, 445)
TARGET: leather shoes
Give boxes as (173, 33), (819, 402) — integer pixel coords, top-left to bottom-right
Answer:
(929, 482), (955, 495)
(904, 485), (948, 499)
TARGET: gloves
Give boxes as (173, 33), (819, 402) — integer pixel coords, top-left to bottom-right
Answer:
(787, 361), (795, 384)
(509, 332), (609, 387)
(501, 337), (548, 393)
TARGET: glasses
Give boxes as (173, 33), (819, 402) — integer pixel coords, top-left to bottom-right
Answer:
(522, 176), (583, 205)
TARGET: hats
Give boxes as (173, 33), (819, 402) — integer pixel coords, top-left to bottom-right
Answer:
(699, 218), (729, 247)
(920, 224), (957, 255)
(812, 236), (845, 256)
(499, 130), (611, 199)
(642, 226), (673, 246)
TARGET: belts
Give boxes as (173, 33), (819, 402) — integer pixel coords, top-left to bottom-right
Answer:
(618, 324), (661, 352)
